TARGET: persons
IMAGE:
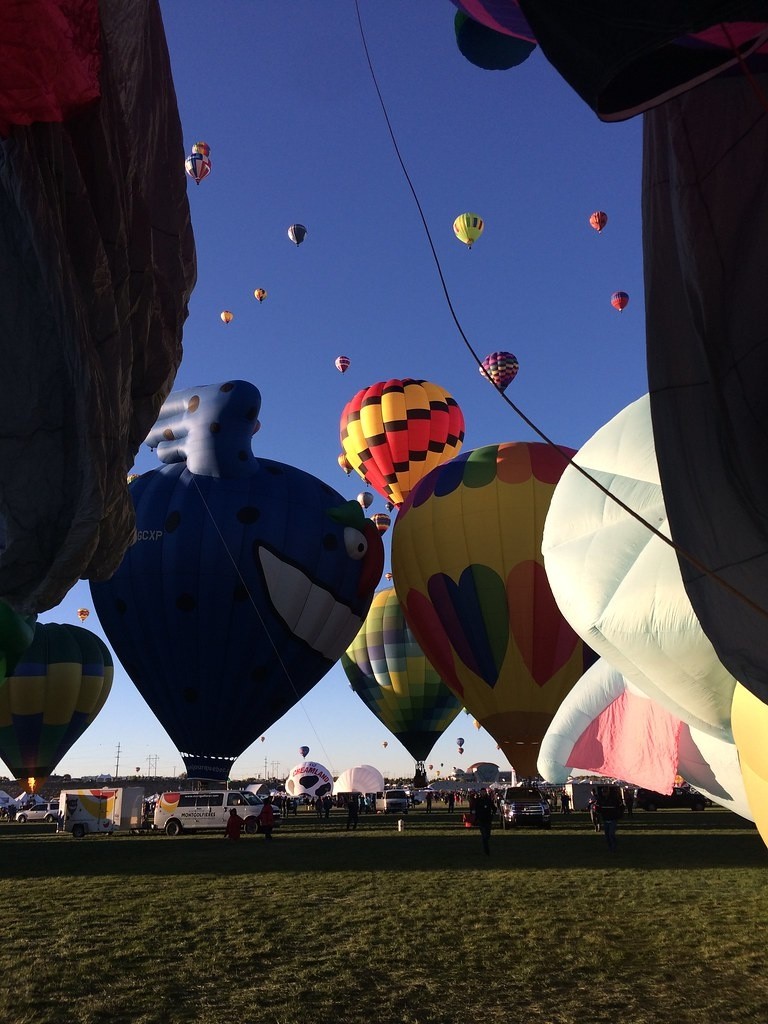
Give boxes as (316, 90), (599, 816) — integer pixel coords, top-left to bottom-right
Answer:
(474, 788), (496, 855)
(255, 798), (274, 842)
(0, 795), (36, 822)
(599, 787), (623, 852)
(542, 788), (571, 815)
(626, 795), (633, 817)
(268, 788), (502, 821)
(348, 796), (359, 830)
(226, 809), (247, 845)
(586, 788), (601, 833)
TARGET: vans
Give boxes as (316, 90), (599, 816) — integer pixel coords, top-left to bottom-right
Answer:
(153, 789), (281, 836)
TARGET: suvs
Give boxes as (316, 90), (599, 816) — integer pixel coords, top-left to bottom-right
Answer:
(375, 790), (408, 814)
(496, 788), (551, 830)
(16, 802), (59, 823)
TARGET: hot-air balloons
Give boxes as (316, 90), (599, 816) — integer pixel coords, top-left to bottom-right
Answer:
(590, 211), (607, 233)
(287, 224), (307, 247)
(185, 140), (211, 186)
(0, 623), (115, 804)
(286, 761), (386, 801)
(335, 356), (350, 375)
(254, 289), (266, 303)
(611, 292), (629, 313)
(479, 351), (519, 396)
(221, 311), (233, 324)
(90, 453), (385, 791)
(77, 608), (89, 623)
(453, 211), (485, 250)
(339, 378), (465, 513)
(370, 513), (392, 536)
(340, 441), (600, 788)
